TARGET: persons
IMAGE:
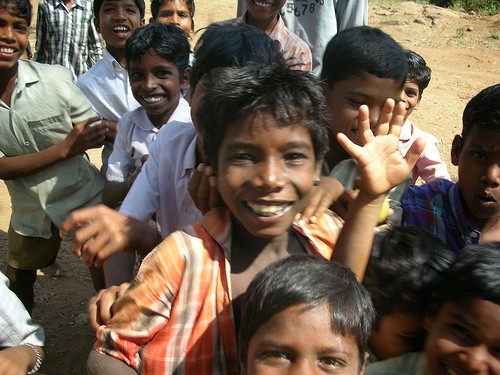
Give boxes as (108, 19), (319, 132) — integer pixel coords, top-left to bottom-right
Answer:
(188, 25), (408, 228)
(237, 254), (377, 375)
(85, 50), (345, 375)
(0, 271), (45, 375)
(329, 49), (500, 375)
(0, 0), (368, 317)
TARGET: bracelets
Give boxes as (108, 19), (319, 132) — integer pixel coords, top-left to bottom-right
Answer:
(143, 225), (159, 247)
(25, 342), (42, 375)
